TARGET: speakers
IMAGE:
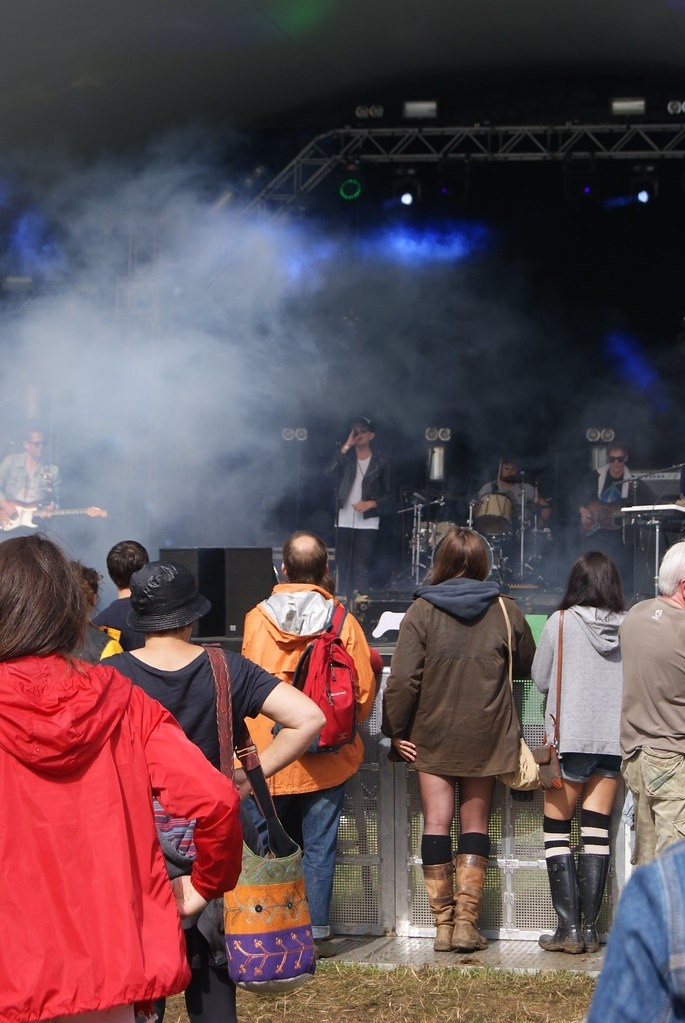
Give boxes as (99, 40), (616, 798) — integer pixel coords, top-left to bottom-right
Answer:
(626, 469), (682, 505)
(159, 545), (274, 637)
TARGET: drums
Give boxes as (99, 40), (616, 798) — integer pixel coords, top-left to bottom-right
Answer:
(428, 520), (457, 551)
(430, 532), (495, 581)
(474, 492), (515, 534)
(409, 520), (437, 553)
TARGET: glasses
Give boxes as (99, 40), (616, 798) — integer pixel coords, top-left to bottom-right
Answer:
(29, 439), (46, 449)
(608, 456), (625, 463)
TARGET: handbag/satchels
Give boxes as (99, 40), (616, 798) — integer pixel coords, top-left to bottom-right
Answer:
(221, 817), (315, 993)
(532, 743), (563, 791)
(494, 736), (543, 791)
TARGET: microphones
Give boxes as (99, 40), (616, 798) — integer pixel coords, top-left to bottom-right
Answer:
(351, 425), (359, 438)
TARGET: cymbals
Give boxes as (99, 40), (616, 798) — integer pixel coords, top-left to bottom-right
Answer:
(500, 472), (537, 483)
(515, 503), (550, 507)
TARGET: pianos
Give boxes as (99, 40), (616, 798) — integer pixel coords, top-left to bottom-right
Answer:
(618, 503), (685, 599)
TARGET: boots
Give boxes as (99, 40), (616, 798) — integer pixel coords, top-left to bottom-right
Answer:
(577, 853), (611, 952)
(452, 854), (489, 951)
(421, 861), (456, 952)
(538, 853), (584, 954)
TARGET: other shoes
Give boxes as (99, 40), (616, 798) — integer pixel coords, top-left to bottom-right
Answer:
(314, 939), (337, 960)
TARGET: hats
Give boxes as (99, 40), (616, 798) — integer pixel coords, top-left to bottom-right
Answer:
(125, 559), (211, 633)
(350, 411), (377, 432)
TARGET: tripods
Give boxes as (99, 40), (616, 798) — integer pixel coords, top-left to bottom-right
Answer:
(506, 476), (552, 590)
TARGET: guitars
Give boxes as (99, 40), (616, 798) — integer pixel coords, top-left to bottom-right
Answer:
(581, 498), (629, 535)
(0, 499), (109, 531)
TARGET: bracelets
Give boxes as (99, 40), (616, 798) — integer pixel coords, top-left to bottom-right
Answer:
(345, 446), (351, 449)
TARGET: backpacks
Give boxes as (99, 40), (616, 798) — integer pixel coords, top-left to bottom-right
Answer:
(271, 604), (364, 755)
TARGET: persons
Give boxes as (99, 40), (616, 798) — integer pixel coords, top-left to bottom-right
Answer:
(478, 455), (552, 593)
(531, 552), (629, 953)
(92, 541), (149, 651)
(323, 414), (397, 596)
(586, 838), (685, 1023)
(0, 430), (62, 541)
(94, 560), (327, 1023)
(75, 566), (123, 663)
(0, 537), (244, 1021)
(569, 442), (633, 561)
(619, 540), (685, 868)
(243, 531), (376, 957)
(381, 531), (535, 951)
